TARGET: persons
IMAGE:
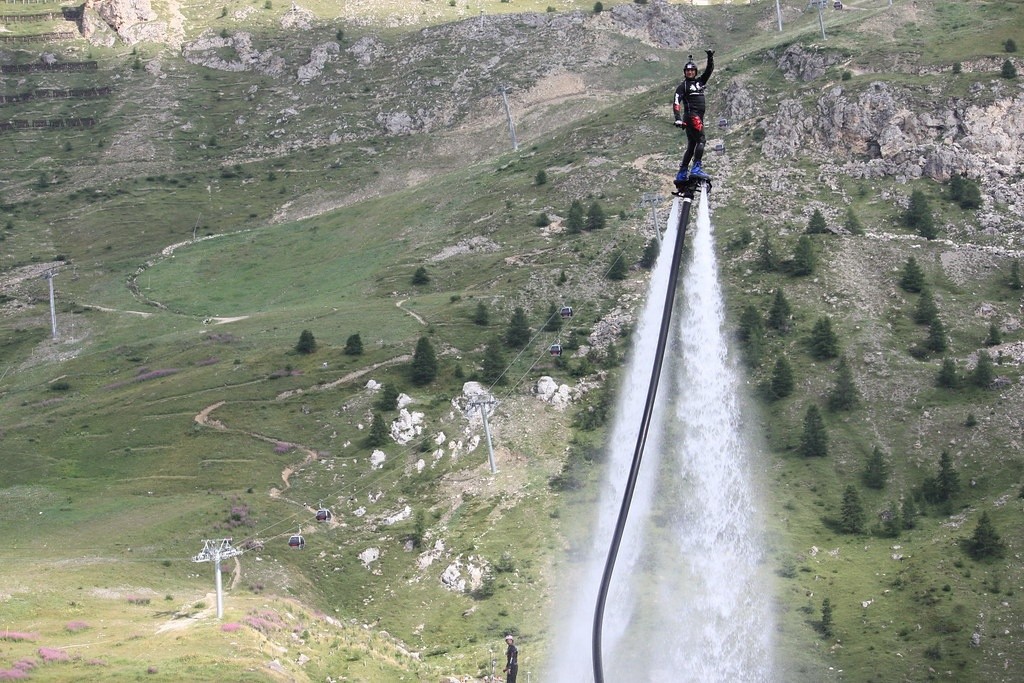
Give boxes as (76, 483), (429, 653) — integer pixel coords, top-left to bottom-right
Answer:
(503, 635), (519, 683)
(673, 49), (716, 185)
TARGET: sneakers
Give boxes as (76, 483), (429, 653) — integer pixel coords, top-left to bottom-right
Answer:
(691, 162), (709, 180)
(676, 169), (688, 183)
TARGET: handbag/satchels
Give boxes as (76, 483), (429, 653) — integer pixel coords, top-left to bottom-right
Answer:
(681, 112), (703, 132)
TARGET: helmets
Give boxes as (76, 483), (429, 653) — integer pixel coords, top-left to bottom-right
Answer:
(684, 55), (697, 76)
(505, 635), (513, 640)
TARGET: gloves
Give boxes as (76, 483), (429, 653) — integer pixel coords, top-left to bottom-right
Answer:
(673, 120), (686, 130)
(704, 50), (715, 57)
